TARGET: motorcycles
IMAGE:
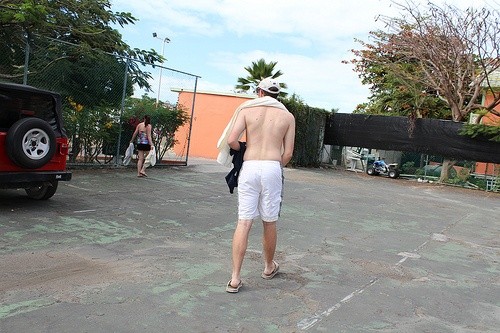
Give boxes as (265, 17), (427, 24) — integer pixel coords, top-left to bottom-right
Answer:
(367, 159), (401, 178)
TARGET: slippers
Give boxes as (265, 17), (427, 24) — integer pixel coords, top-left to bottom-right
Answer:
(226, 279), (242, 293)
(137, 171), (147, 178)
(262, 261), (279, 279)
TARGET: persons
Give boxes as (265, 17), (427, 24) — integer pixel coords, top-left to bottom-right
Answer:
(226, 79), (296, 292)
(130, 115), (154, 177)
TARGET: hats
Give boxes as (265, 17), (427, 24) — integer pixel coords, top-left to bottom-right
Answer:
(256, 76), (280, 93)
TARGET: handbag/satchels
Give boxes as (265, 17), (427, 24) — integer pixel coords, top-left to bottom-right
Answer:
(144, 146), (157, 167)
(123, 143), (133, 166)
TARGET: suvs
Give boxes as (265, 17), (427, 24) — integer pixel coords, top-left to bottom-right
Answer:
(0, 80), (73, 199)
